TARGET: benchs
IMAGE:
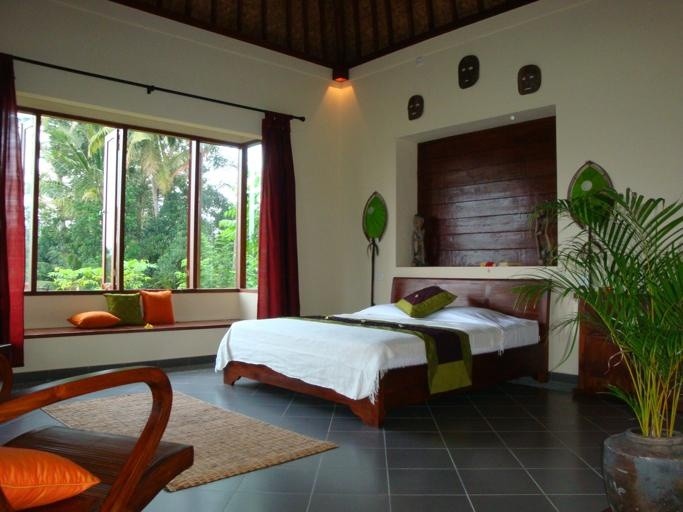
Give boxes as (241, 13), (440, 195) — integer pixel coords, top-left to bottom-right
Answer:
(9, 317), (247, 375)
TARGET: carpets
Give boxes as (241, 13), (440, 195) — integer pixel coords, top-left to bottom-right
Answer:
(38, 385), (340, 494)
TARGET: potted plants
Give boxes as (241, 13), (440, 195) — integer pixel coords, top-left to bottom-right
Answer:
(503, 183), (683, 511)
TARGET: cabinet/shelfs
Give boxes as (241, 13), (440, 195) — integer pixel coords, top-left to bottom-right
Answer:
(567, 290), (682, 414)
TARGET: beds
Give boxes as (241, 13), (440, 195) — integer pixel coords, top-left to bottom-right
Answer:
(213, 275), (555, 429)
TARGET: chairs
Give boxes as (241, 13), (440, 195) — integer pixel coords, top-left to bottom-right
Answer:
(0, 342), (195, 512)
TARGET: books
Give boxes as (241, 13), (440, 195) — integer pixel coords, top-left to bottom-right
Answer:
(499, 262), (522, 266)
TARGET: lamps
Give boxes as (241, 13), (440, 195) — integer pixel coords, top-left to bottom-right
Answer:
(359, 189), (389, 310)
(330, 64), (351, 83)
(565, 159), (614, 290)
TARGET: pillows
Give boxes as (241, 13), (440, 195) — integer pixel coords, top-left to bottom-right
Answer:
(357, 298), (417, 320)
(392, 282), (459, 320)
(431, 304), (505, 323)
(66, 308), (123, 330)
(100, 291), (147, 326)
(138, 286), (178, 326)
